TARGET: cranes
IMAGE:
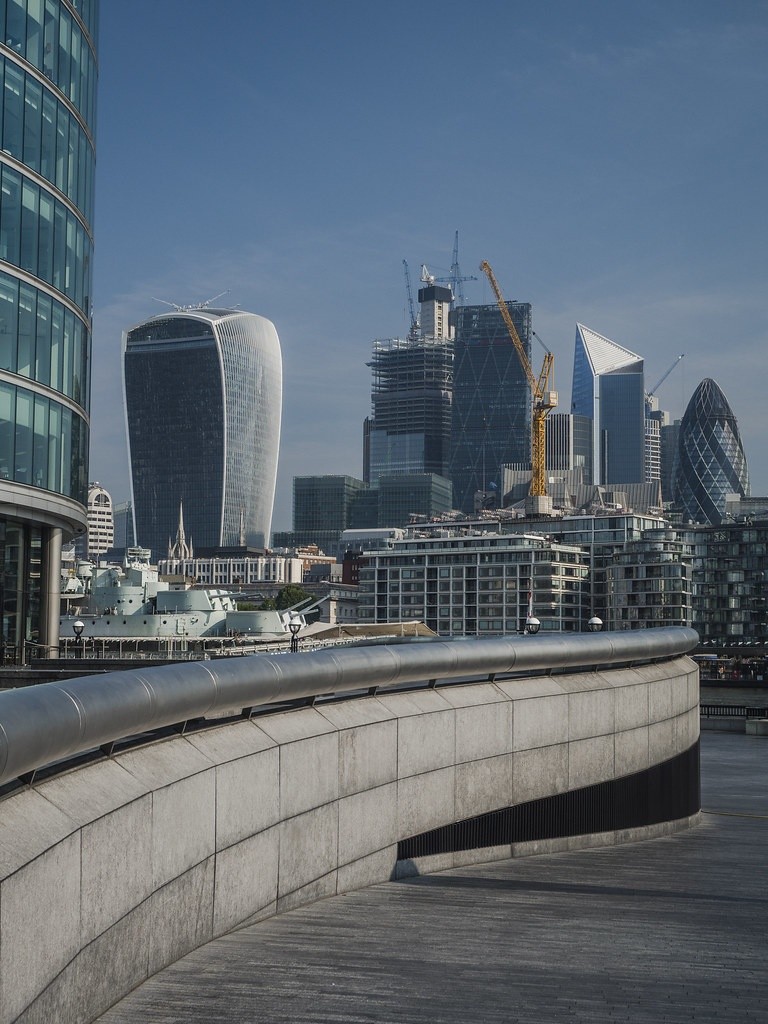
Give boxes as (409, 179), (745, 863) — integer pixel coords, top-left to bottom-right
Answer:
(478, 257), (560, 518)
(434, 230), (476, 308)
(399, 259), (420, 343)
